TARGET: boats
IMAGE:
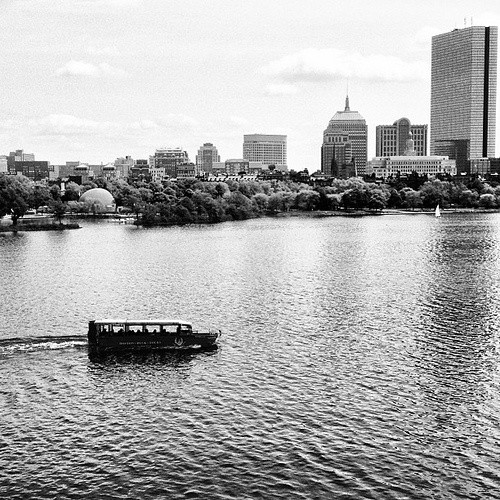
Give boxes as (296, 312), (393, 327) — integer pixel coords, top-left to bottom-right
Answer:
(87, 319), (222, 359)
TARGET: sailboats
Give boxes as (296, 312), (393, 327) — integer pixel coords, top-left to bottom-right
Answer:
(434, 203), (441, 219)
